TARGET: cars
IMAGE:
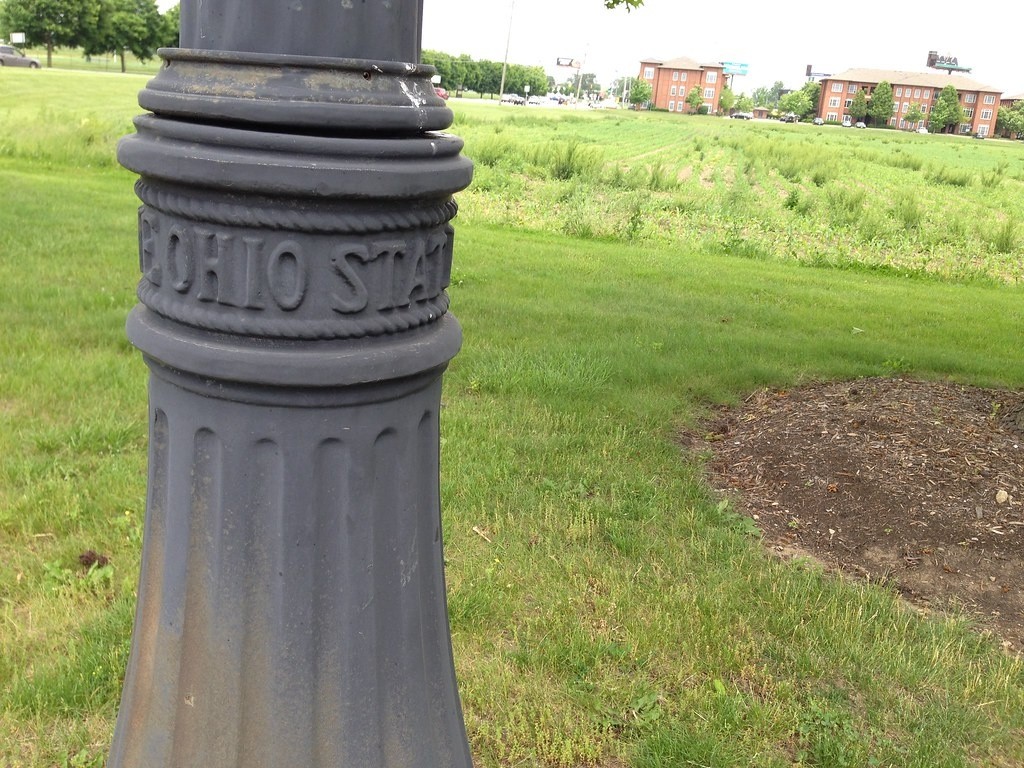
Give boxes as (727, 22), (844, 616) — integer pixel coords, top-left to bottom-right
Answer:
(855, 121), (867, 128)
(434, 87), (449, 100)
(813, 117), (825, 126)
(730, 112), (755, 122)
(973, 133), (984, 139)
(501, 92), (576, 106)
(0, 45), (41, 69)
(916, 127), (928, 134)
(841, 121), (852, 127)
(780, 114), (799, 123)
